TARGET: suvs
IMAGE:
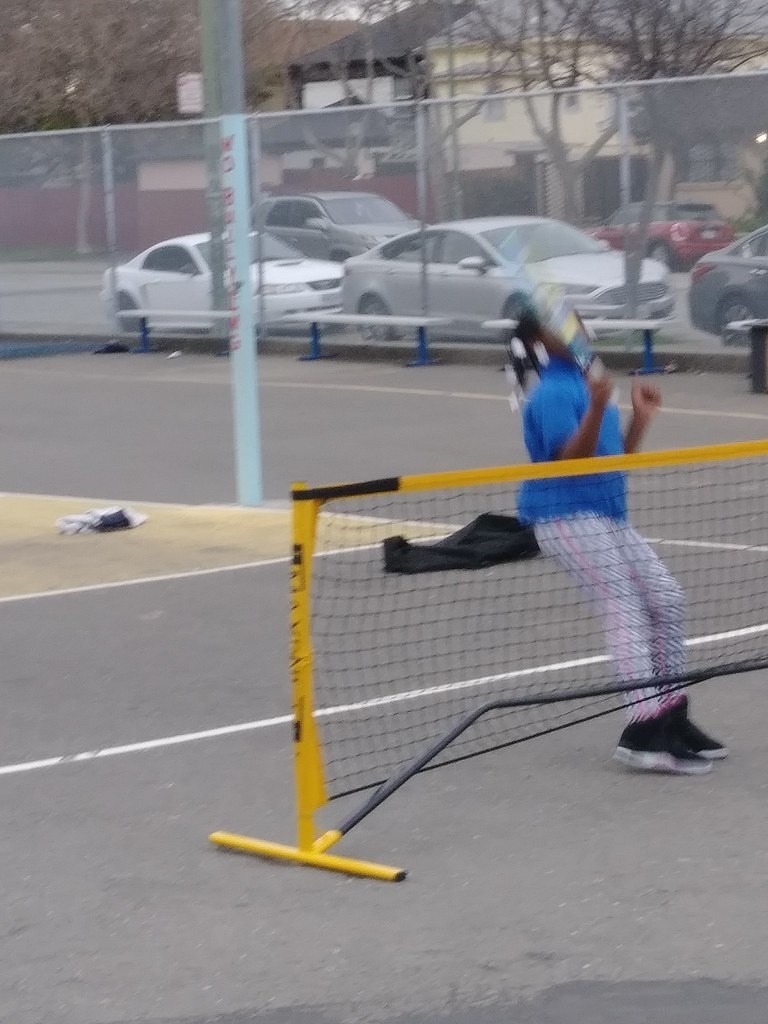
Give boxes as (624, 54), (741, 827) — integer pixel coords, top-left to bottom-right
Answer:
(252, 191), (427, 262)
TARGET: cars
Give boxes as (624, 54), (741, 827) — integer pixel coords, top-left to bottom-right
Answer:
(102, 228), (347, 351)
(689, 224), (768, 346)
(343, 216), (676, 349)
(585, 202), (735, 271)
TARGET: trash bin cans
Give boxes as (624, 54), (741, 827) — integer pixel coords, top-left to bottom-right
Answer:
(748, 322), (768, 392)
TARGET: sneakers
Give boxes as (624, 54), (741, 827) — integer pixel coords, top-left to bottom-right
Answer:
(664, 696), (728, 759)
(613, 716), (712, 775)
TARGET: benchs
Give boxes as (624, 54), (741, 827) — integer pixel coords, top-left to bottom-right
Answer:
(118, 309), (232, 353)
(284, 312), (452, 367)
(481, 316), (671, 376)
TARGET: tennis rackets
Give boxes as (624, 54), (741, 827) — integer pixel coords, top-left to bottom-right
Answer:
(483, 226), (624, 412)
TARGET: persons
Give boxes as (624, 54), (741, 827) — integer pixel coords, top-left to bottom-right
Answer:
(508, 300), (727, 775)
